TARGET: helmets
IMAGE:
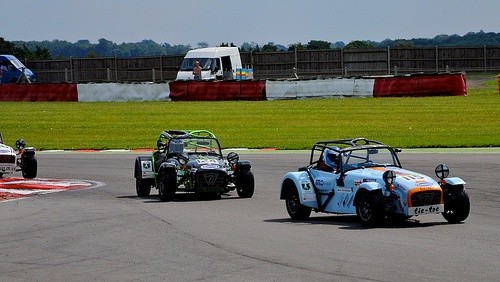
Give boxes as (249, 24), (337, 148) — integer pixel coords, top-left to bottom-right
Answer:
(323, 146), (346, 168)
(169, 138), (183, 153)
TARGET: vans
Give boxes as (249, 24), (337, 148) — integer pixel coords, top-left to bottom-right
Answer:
(175, 47), (242, 80)
(0, 54), (36, 84)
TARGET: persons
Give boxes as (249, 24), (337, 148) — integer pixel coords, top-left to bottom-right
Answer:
(192, 60), (204, 80)
(166, 138), (191, 162)
(315, 145), (356, 175)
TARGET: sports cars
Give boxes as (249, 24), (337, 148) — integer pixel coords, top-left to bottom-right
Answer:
(0, 133), (37, 180)
(135, 129), (254, 201)
(278, 136), (470, 225)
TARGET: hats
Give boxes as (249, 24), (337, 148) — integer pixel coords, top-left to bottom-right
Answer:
(195, 61), (199, 63)
